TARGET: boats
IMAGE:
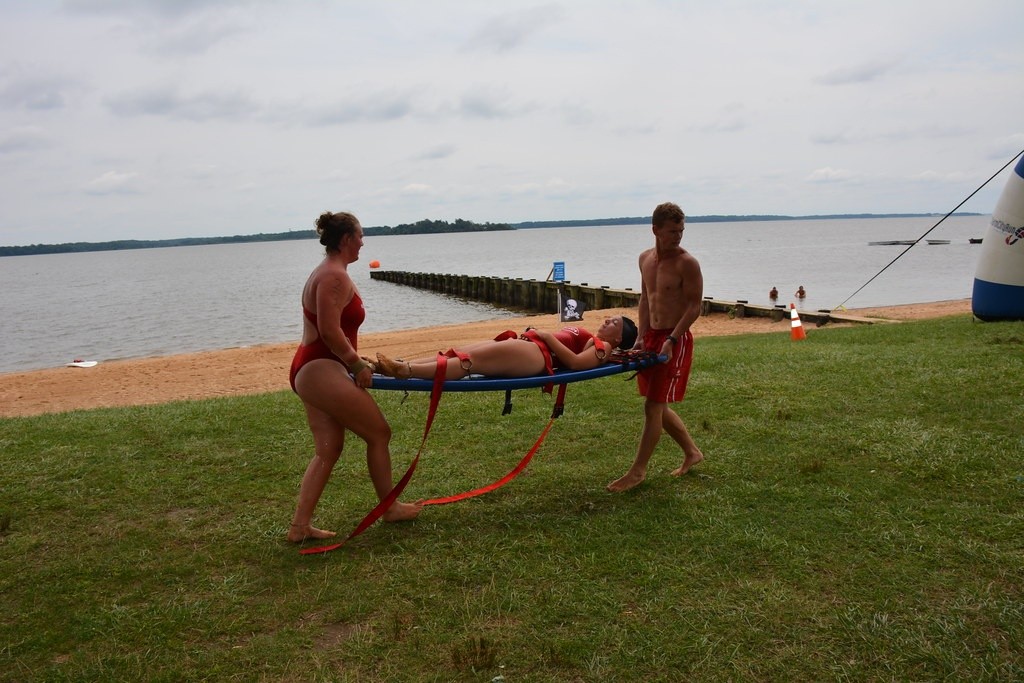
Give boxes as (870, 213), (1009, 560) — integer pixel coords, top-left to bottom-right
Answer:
(969, 238), (983, 244)
(926, 239), (951, 244)
(66, 360), (97, 368)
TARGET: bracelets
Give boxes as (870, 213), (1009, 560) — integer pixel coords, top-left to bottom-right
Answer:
(666, 336), (677, 344)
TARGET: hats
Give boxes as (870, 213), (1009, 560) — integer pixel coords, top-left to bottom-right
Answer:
(617, 316), (638, 350)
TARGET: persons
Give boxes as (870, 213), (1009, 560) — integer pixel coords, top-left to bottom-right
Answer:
(360, 315), (638, 381)
(794, 285), (807, 301)
(607, 202), (729, 495)
(769, 286), (779, 302)
(287, 211), (426, 544)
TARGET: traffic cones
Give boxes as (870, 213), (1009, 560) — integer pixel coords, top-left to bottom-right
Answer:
(790, 304), (805, 341)
